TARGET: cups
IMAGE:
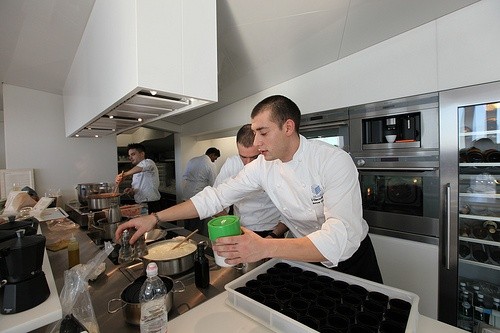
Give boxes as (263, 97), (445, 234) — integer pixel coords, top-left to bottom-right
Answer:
(234, 262), (411, 333)
(385, 135), (397, 143)
(208, 214), (244, 268)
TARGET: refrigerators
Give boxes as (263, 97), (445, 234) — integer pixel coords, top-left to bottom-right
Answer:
(437, 81), (500, 333)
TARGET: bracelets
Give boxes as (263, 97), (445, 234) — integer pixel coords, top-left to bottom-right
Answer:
(267, 230), (279, 239)
(150, 212), (161, 226)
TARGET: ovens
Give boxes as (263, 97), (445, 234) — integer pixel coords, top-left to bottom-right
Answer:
(348, 93), (441, 238)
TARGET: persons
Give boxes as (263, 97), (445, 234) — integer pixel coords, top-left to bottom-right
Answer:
(210, 123), (289, 256)
(181, 146), (227, 238)
(111, 142), (162, 219)
(116, 94), (385, 286)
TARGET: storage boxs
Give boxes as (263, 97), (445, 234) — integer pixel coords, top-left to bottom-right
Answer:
(224, 257), (420, 333)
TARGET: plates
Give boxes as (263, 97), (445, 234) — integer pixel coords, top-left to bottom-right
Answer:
(397, 139), (416, 143)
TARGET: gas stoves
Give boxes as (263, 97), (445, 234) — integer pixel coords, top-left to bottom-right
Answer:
(65, 200), (124, 227)
(86, 224), (215, 287)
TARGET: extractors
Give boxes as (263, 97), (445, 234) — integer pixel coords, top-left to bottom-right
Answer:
(72, 90), (191, 139)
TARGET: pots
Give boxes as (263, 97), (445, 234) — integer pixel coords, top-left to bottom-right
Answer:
(75, 182), (198, 275)
(0, 216), (50, 315)
(107, 275), (186, 324)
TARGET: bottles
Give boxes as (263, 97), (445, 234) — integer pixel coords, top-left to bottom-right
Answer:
(194, 239), (210, 289)
(458, 146), (500, 331)
(108, 200), (120, 224)
(139, 263), (168, 333)
(117, 229), (135, 264)
(132, 230), (148, 257)
(69, 232), (80, 269)
